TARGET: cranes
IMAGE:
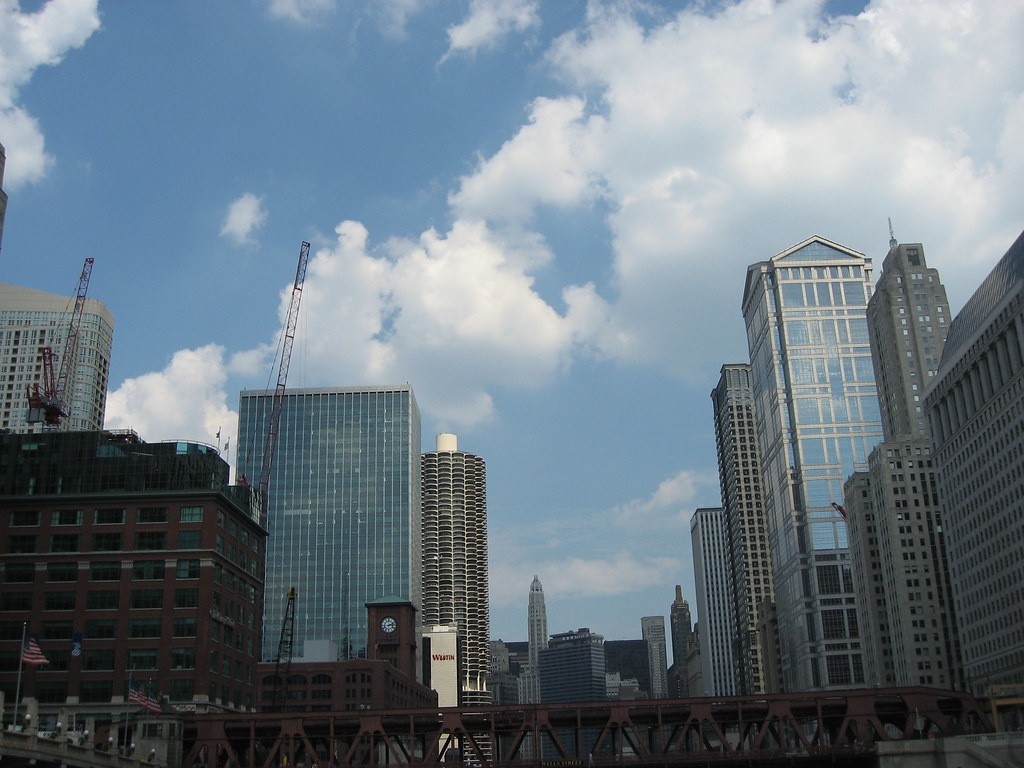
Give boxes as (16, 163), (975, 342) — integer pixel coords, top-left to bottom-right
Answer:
(238, 239), (312, 525)
(23, 256), (95, 434)
(270, 586), (296, 713)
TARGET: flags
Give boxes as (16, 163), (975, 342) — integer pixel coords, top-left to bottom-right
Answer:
(22, 626), (50, 664)
(129, 671), (147, 706)
(225, 442), (228, 450)
(237, 475), (248, 487)
(216, 431), (221, 438)
(145, 686), (162, 713)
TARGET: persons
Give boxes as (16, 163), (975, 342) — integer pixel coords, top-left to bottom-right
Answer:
(615, 753), (620, 762)
(855, 736), (864, 752)
(588, 752), (594, 767)
